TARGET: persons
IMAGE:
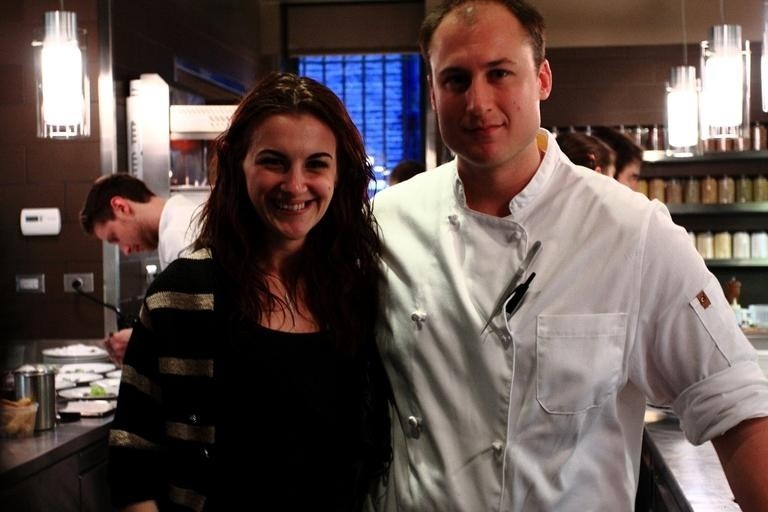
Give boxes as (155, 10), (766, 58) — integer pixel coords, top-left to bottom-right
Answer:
(387, 160), (426, 188)
(106, 73), (396, 512)
(555, 130), (616, 180)
(590, 123), (644, 192)
(361, 0), (768, 512)
(80, 172), (211, 369)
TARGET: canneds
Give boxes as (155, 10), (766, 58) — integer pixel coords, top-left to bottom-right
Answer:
(547, 122), (768, 260)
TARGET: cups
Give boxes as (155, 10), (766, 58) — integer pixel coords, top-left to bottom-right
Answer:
(634, 172), (767, 204)
(686, 228), (768, 260)
(10, 367), (58, 432)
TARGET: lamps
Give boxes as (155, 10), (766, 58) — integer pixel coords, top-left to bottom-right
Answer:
(34, 0), (92, 141)
(665, 0), (768, 157)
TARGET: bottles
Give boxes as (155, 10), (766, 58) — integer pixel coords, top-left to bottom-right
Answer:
(623, 121), (766, 151)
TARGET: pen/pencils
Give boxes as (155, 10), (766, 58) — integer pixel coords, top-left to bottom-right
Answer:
(506, 273), (536, 318)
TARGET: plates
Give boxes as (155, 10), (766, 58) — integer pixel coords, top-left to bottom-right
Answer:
(40, 341), (126, 417)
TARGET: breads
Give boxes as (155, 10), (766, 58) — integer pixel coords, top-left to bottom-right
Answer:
(1, 398), (37, 434)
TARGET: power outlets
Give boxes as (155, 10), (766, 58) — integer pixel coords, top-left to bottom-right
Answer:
(63, 273), (95, 294)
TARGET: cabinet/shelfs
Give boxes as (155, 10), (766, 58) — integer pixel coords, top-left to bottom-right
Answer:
(638, 151), (768, 268)
